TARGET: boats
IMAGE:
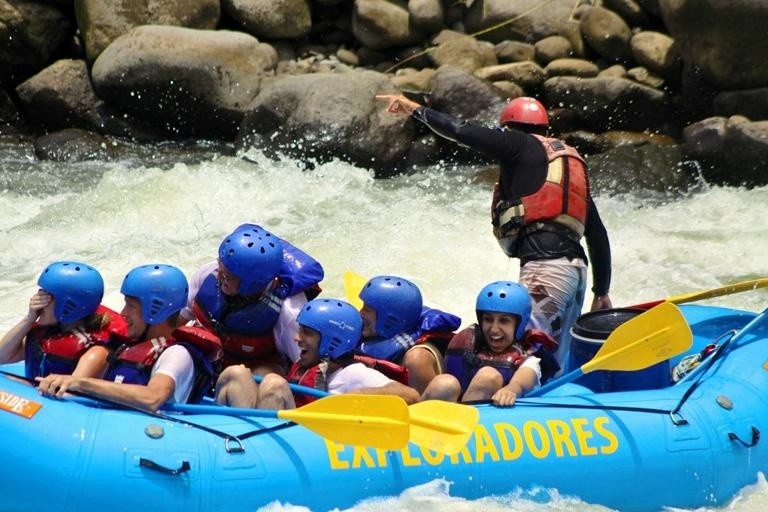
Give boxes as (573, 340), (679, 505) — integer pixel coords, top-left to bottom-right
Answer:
(1, 298), (768, 512)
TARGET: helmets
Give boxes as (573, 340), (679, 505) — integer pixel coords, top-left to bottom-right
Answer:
(477, 281), (532, 341)
(219, 224), (282, 292)
(38, 262), (104, 322)
(121, 265), (188, 326)
(499, 97), (549, 126)
(296, 277), (422, 359)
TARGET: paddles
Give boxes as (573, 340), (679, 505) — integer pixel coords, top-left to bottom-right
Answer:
(163, 394), (411, 450)
(524, 302), (693, 399)
(344, 269), (367, 314)
(253, 375), (478, 456)
(630, 279), (767, 310)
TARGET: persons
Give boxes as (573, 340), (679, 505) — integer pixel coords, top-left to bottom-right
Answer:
(373, 90), (613, 383)
(34, 263), (206, 410)
(1, 260), (118, 384)
(213, 297), (421, 416)
(177, 216), (326, 374)
(418, 278), (560, 406)
(356, 273), (462, 397)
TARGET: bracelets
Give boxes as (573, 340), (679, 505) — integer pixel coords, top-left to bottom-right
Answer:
(595, 294), (609, 299)
